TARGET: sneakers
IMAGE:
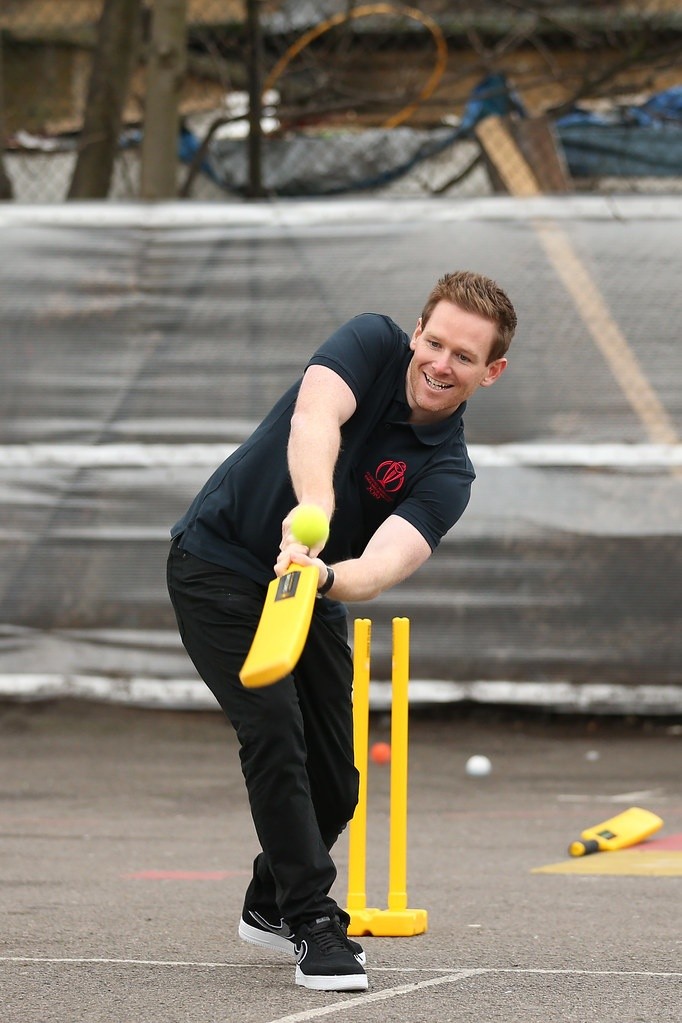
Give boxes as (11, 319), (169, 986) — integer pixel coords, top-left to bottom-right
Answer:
(295, 916), (369, 994)
(239, 903), (365, 964)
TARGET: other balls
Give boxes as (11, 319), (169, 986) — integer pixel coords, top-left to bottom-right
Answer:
(464, 752), (493, 780)
(370, 741), (392, 764)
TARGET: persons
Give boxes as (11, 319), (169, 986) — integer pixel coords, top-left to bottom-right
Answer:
(166, 270), (518, 990)
